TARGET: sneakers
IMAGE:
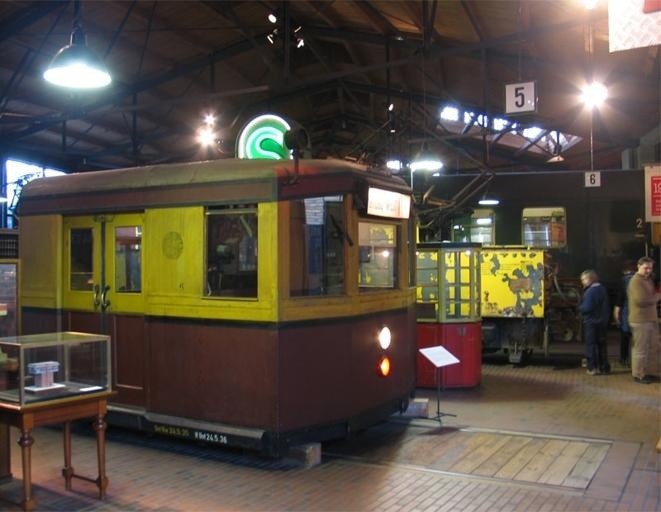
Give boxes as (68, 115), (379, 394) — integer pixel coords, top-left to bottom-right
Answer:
(587, 367), (610, 375)
(621, 358), (660, 384)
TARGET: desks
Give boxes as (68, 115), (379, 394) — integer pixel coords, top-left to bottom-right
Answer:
(1, 392), (120, 511)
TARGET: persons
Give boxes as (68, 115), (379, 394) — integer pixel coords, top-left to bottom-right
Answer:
(626, 257), (661, 384)
(577, 269), (611, 375)
(613, 260), (638, 365)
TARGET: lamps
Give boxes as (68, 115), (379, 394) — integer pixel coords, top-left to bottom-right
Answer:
(38, 2), (113, 88)
(282, 127), (310, 187)
(406, 0), (445, 172)
